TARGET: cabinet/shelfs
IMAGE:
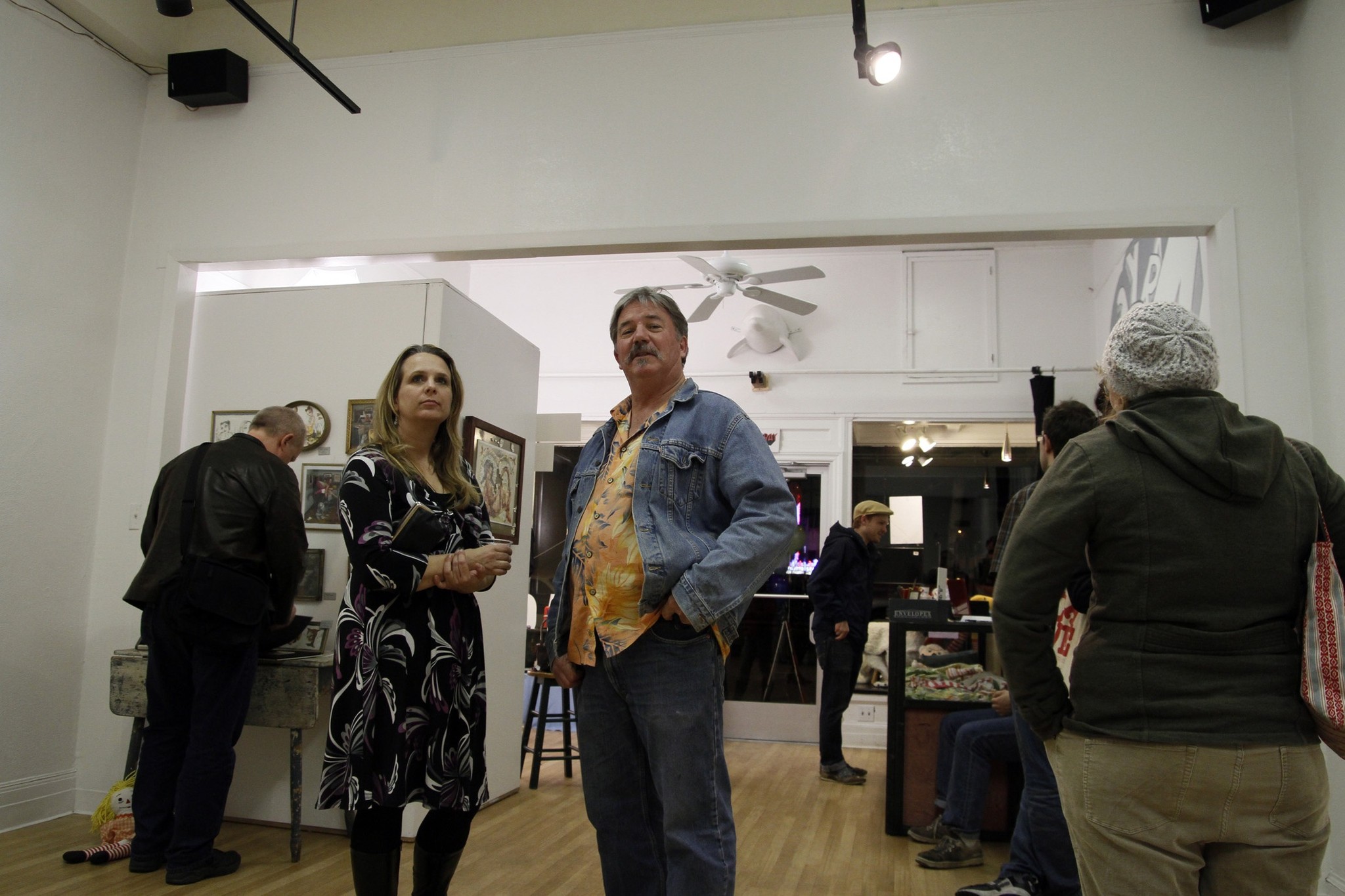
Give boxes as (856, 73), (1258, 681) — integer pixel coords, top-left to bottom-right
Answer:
(884, 621), (1013, 842)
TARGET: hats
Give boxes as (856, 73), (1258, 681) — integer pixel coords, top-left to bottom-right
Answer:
(854, 501), (894, 520)
(1102, 302), (1220, 399)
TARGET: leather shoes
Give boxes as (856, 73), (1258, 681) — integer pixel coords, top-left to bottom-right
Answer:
(821, 768), (866, 784)
(850, 768), (868, 774)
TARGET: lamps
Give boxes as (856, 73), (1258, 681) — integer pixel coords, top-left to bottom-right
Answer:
(999, 427), (1013, 463)
(983, 469), (992, 490)
(725, 303), (804, 361)
(917, 425), (935, 454)
(891, 423), (917, 453)
(853, 40), (903, 87)
(917, 453), (933, 468)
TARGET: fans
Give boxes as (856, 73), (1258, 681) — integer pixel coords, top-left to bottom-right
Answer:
(614, 254), (826, 323)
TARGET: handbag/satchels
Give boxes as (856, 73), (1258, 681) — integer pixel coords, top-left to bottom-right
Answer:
(1288, 439), (1345, 755)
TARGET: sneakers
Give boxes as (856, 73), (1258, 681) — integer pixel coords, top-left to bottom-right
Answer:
(909, 815), (948, 843)
(916, 835), (983, 868)
(167, 851), (241, 883)
(955, 878), (1035, 896)
(129, 854), (164, 872)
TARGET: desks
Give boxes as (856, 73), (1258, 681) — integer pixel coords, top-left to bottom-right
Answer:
(108, 648), (332, 864)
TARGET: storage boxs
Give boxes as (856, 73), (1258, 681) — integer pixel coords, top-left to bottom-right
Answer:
(886, 597), (954, 623)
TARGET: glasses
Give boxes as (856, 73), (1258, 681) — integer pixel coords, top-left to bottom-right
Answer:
(1036, 435), (1044, 442)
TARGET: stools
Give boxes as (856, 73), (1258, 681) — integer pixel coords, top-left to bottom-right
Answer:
(519, 669), (581, 790)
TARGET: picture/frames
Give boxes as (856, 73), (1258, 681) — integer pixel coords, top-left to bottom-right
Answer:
(274, 621), (331, 653)
(286, 400), (331, 453)
(294, 548), (324, 602)
(299, 462), (346, 532)
(463, 415), (527, 545)
(346, 399), (375, 454)
(210, 410), (259, 442)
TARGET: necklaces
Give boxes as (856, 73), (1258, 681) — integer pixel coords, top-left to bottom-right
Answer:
(410, 459), (445, 494)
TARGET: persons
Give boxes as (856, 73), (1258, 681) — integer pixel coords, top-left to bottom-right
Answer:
(120, 406), (308, 886)
(540, 285), (800, 896)
(806, 500), (893, 785)
(907, 377), (1116, 896)
(991, 301), (1344, 896)
(315, 344), (513, 896)
